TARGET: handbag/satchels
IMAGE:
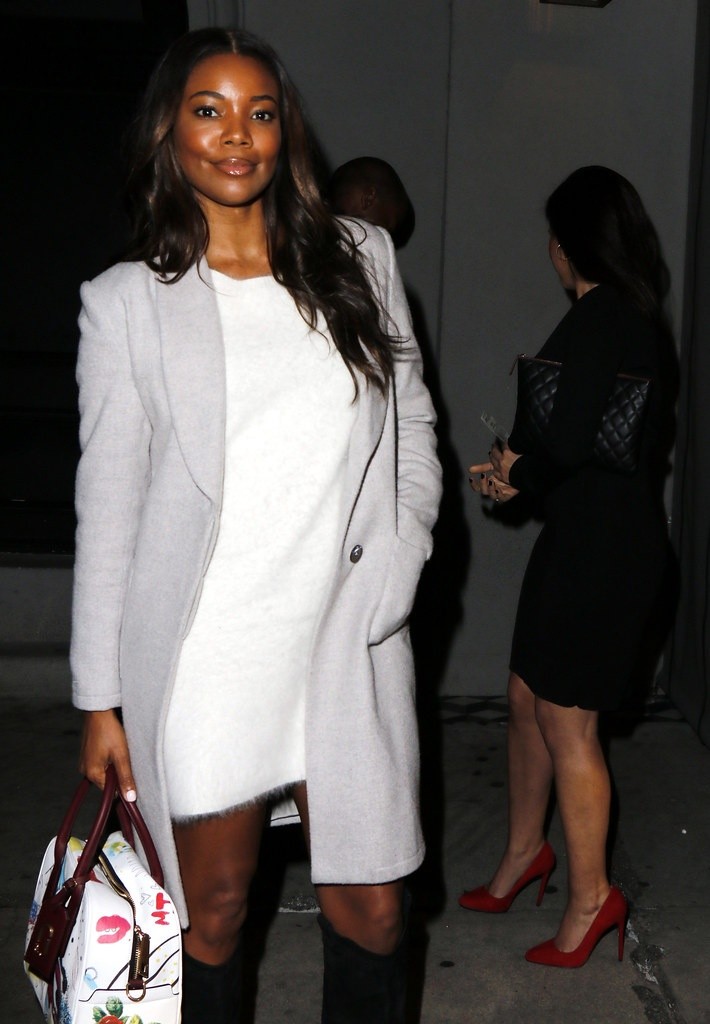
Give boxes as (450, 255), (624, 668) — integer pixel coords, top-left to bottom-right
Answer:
(506, 352), (653, 477)
(23, 759), (184, 1024)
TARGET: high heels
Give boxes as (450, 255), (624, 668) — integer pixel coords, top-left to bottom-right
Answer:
(459, 839), (555, 914)
(525, 886), (626, 968)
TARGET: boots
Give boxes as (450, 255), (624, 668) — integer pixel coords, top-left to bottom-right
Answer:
(317, 884), (415, 1024)
(180, 928), (244, 1024)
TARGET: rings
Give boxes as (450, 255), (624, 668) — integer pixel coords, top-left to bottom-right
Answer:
(495, 499), (499, 502)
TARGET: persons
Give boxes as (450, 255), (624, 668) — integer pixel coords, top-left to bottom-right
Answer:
(458, 166), (673, 968)
(69, 23), (442, 1024)
